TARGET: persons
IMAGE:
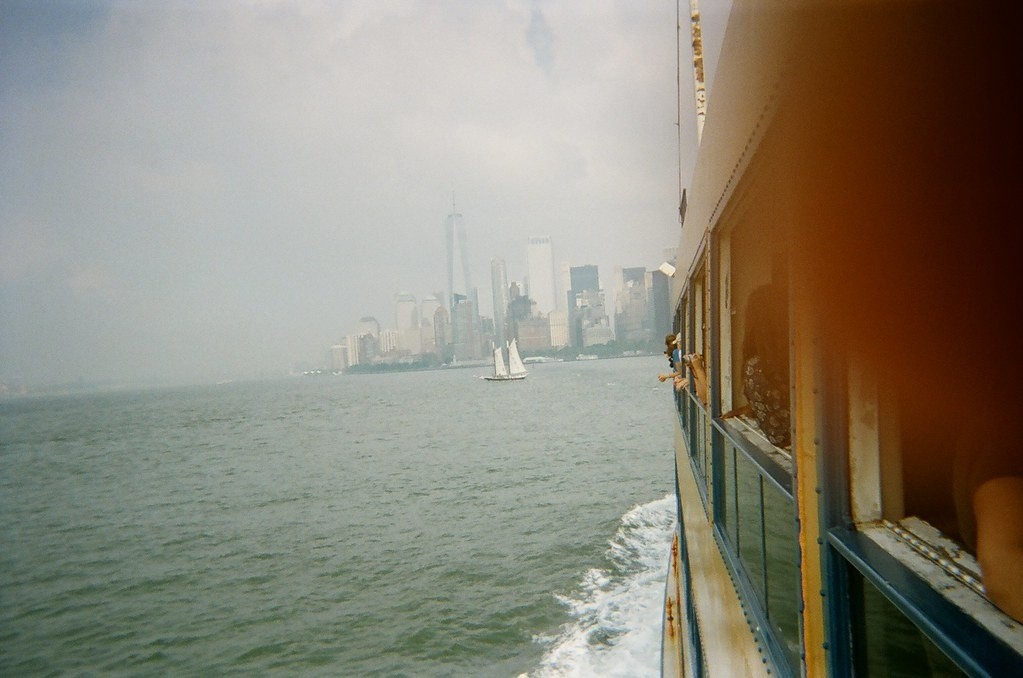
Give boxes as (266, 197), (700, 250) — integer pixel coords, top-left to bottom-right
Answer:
(966, 287), (1022, 626)
(657, 276), (798, 452)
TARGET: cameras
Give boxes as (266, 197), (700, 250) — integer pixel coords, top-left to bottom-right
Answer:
(682, 354), (696, 364)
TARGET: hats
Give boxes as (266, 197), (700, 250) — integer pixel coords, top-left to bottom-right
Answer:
(671, 332), (680, 345)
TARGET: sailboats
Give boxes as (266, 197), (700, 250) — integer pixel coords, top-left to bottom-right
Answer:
(484, 337), (529, 382)
